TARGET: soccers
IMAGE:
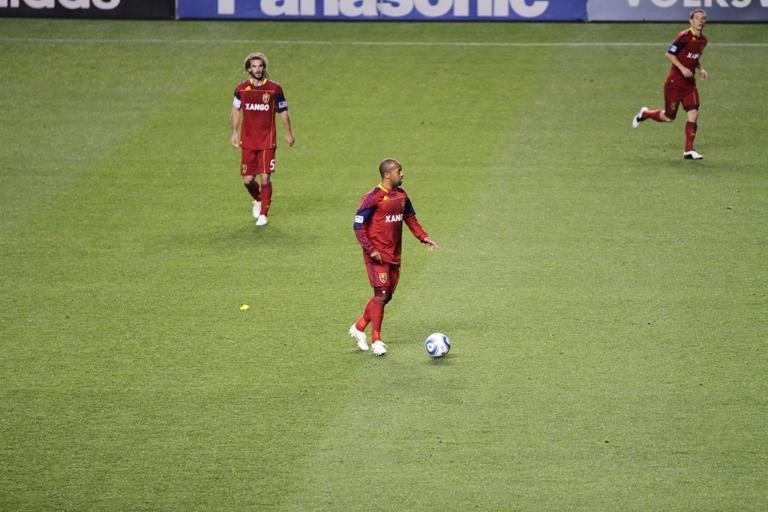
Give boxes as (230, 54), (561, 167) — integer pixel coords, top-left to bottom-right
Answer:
(425, 332), (451, 358)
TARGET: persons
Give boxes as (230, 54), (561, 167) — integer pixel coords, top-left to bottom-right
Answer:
(630, 8), (711, 160)
(229, 51), (296, 227)
(346, 159), (437, 358)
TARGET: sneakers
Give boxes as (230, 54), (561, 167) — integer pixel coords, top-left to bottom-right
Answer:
(256, 215), (267, 227)
(683, 149), (702, 160)
(632, 107), (647, 129)
(252, 201), (262, 219)
(372, 340), (385, 356)
(349, 322), (368, 351)
